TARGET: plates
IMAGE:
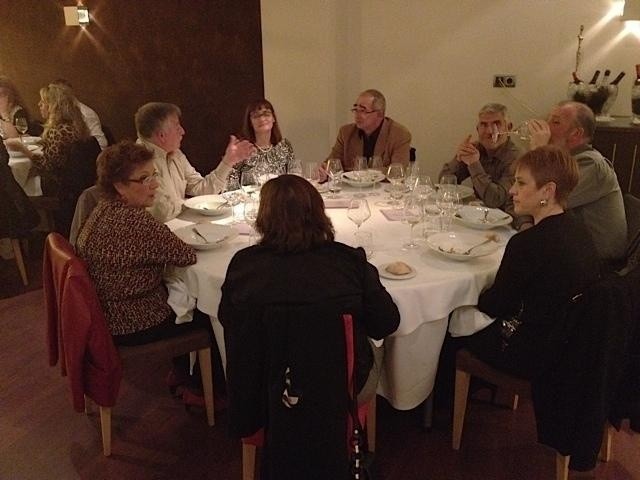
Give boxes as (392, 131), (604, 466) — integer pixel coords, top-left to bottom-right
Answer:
(184, 195), (239, 216)
(378, 264), (416, 280)
(19, 136), (43, 144)
(454, 206), (514, 230)
(7, 144), (39, 156)
(426, 231), (498, 263)
(172, 224), (239, 251)
(415, 184), (475, 204)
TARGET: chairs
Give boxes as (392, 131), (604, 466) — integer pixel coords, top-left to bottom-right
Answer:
(622, 190), (640, 258)
(225, 303), (379, 480)
(0, 164), (29, 287)
(49, 232), (220, 455)
(450, 263), (620, 479)
(33, 136), (100, 254)
(409, 146), (416, 165)
(0, 235), (30, 287)
(70, 185), (102, 251)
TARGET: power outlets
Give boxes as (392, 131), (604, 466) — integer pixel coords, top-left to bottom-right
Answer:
(493, 73), (505, 87)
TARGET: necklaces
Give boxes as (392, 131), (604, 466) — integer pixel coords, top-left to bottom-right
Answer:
(252, 141), (274, 153)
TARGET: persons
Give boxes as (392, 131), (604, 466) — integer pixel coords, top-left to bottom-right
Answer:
(3, 83), (97, 206)
(312, 89), (412, 181)
(218, 174), (400, 424)
(505, 100), (629, 278)
(221, 97), (302, 190)
(75, 138), (232, 413)
(446, 144), (600, 403)
(438, 103), (522, 207)
(132, 101), (256, 222)
(50, 78), (108, 152)
(0, 77), (30, 139)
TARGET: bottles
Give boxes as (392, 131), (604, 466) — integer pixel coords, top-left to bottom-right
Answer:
(571, 69), (625, 115)
(631, 63), (640, 124)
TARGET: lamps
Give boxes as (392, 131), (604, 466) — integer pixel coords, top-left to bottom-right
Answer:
(78, 6), (90, 24)
(620, 0), (640, 22)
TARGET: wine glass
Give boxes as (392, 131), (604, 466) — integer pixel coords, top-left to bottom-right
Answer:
(15, 118), (28, 134)
(221, 156), (463, 259)
(21, 134), (30, 147)
(491, 121), (531, 144)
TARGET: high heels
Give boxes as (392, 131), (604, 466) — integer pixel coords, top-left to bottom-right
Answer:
(181, 385), (232, 415)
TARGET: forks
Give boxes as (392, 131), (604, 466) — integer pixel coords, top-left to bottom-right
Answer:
(192, 227), (212, 245)
(463, 238), (492, 255)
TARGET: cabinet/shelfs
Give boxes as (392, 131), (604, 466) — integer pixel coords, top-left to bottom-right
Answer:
(591, 114), (640, 198)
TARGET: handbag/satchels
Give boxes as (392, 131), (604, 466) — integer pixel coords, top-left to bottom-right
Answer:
(336, 311), (381, 479)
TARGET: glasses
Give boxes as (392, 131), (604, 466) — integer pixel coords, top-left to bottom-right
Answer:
(350, 106), (384, 114)
(249, 111), (274, 119)
(118, 169), (161, 185)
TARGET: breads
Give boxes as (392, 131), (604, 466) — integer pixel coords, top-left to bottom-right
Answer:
(386, 261), (410, 275)
